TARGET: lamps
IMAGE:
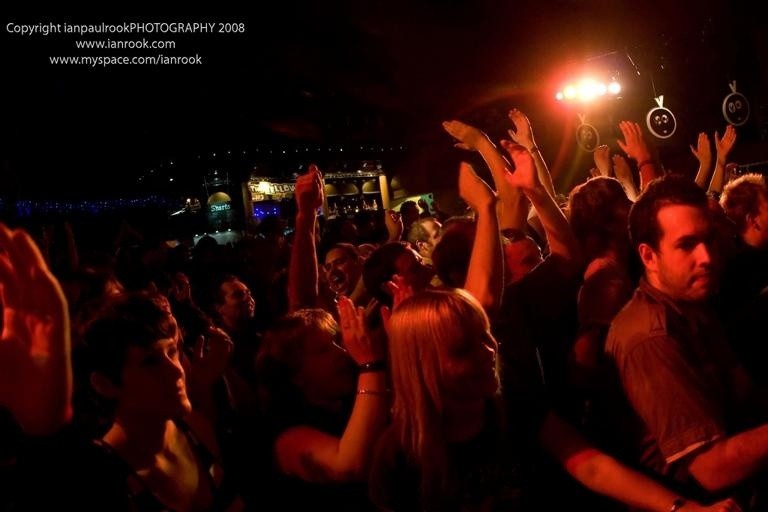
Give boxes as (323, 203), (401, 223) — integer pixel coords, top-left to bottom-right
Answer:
(325, 184), (337, 196)
(207, 192), (231, 204)
(342, 183), (359, 196)
(362, 181), (380, 193)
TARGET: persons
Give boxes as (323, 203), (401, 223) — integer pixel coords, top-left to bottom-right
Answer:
(1, 105), (766, 512)
